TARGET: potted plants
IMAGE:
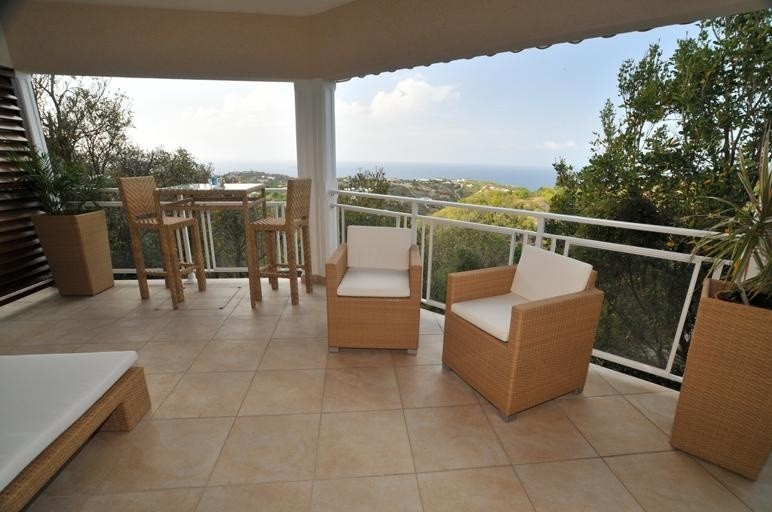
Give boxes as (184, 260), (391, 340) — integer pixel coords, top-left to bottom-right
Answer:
(667, 123), (771, 482)
(3, 142), (116, 299)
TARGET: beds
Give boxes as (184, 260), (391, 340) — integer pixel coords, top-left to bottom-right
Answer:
(2, 351), (152, 510)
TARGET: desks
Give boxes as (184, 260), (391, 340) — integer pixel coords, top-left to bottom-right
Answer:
(153, 180), (281, 309)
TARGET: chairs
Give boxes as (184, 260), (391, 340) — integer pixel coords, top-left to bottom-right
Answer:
(439, 243), (608, 422)
(117, 174), (208, 304)
(324, 222), (423, 356)
(249, 175), (316, 305)
